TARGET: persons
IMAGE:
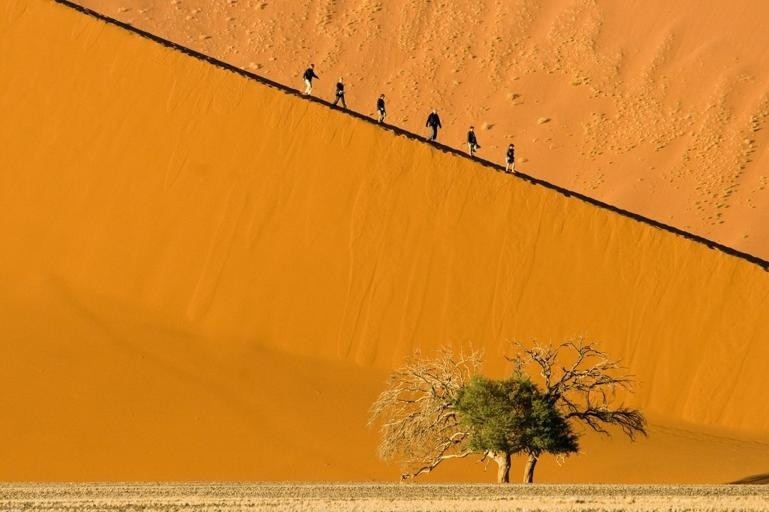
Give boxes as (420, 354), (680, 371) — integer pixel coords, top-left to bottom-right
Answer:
(466, 127), (477, 157)
(333, 77), (347, 108)
(376, 94), (386, 124)
(302, 64), (320, 96)
(506, 144), (517, 174)
(426, 110), (442, 142)
